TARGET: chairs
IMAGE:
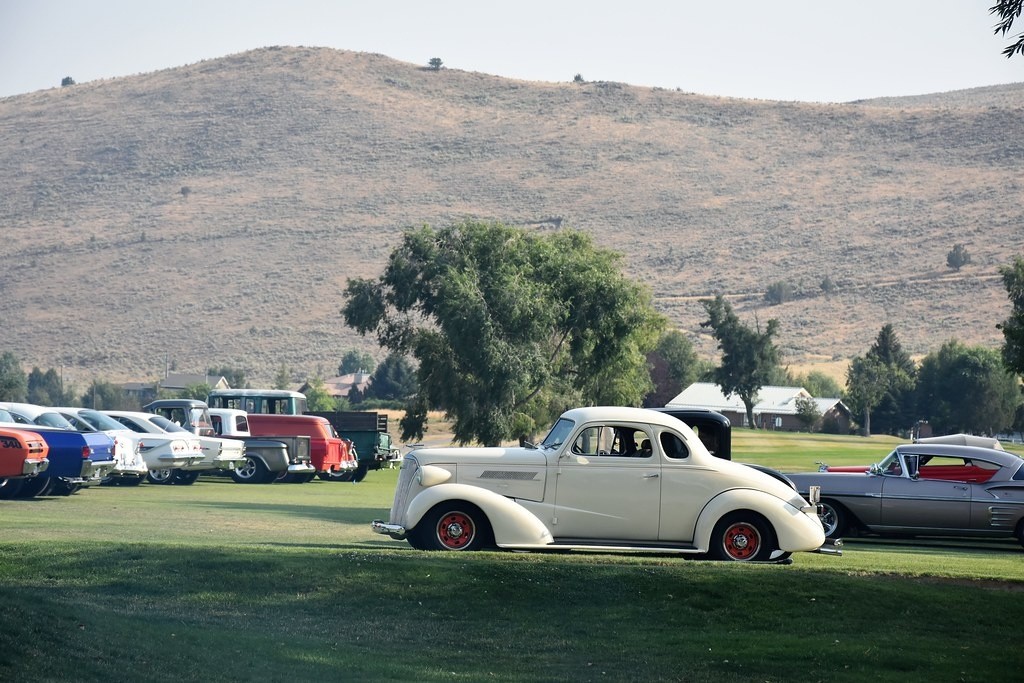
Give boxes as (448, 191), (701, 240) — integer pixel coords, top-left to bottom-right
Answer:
(629, 439), (653, 457)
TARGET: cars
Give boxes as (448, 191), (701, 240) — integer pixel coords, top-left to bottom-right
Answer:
(0, 388), (402, 499)
(580, 406), (733, 461)
(814, 434), (1004, 483)
(780, 443), (1024, 549)
(371, 404), (843, 563)
